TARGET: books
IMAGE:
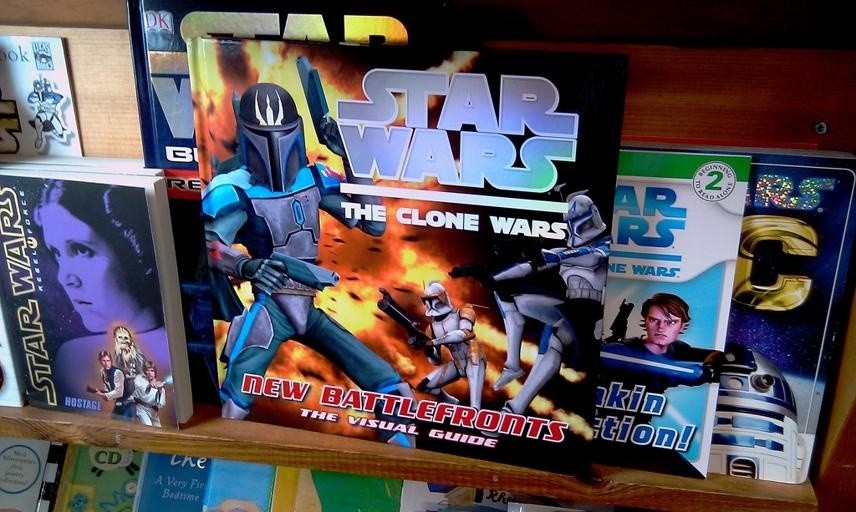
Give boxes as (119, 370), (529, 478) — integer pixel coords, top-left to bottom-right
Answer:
(0, 435), (562, 512)
(676, 140), (854, 484)
(1, 22), (194, 430)
(596, 148), (754, 481)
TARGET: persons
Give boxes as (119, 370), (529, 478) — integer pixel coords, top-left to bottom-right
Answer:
(128, 2), (632, 478)
(600, 289), (724, 479)
